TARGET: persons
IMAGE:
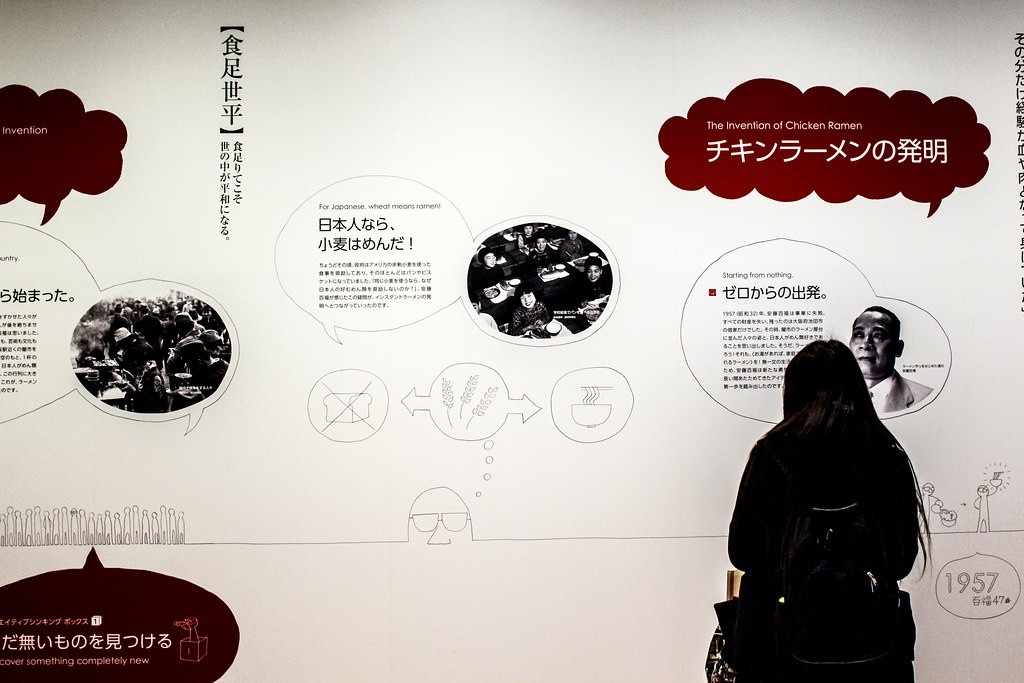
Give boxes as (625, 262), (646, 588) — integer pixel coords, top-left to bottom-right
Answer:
(849, 306), (934, 413)
(74, 290), (230, 413)
(727, 340), (932, 683)
(470, 223), (611, 337)
